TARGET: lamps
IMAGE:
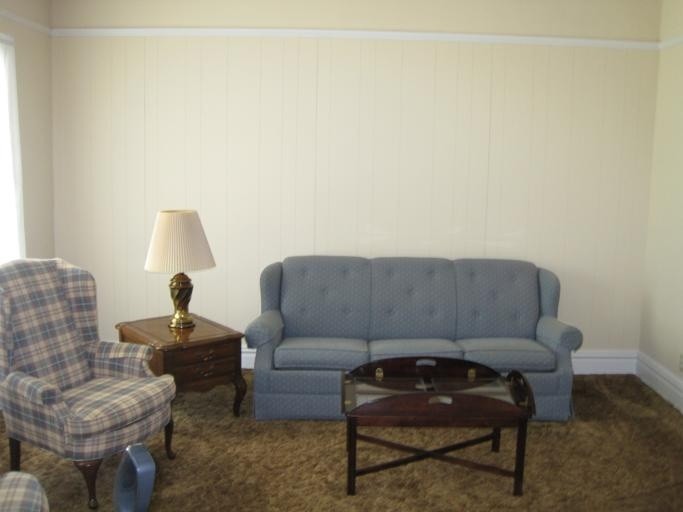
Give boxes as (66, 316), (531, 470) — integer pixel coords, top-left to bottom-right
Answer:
(144, 210), (216, 329)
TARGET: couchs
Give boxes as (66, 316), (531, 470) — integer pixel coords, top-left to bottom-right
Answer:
(245, 256), (582, 423)
